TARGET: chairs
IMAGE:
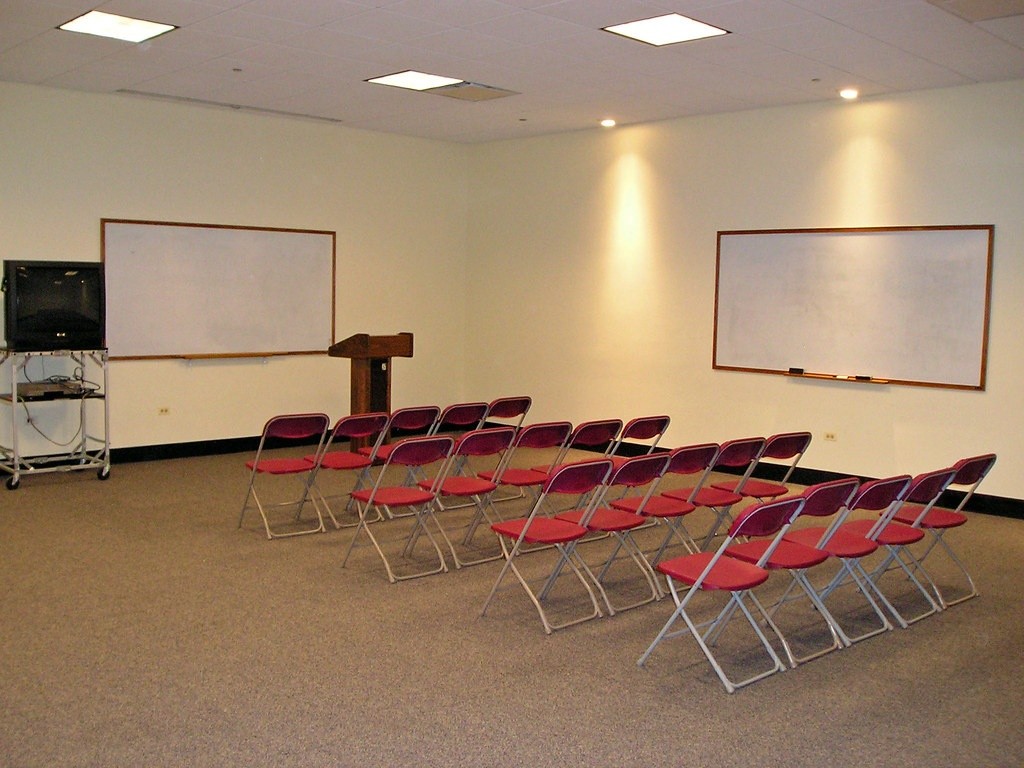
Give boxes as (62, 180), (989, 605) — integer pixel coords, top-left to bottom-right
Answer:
(600, 443), (720, 598)
(589, 415), (671, 537)
(478, 458), (614, 635)
(636, 495), (807, 695)
(867, 454), (997, 611)
(701, 431), (813, 552)
(822, 467), (958, 630)
(711, 476), (861, 670)
(341, 434), (457, 584)
(406, 402), (490, 512)
(530, 419), (624, 545)
(296, 411), (385, 530)
(653, 437), (768, 553)
(542, 452), (672, 617)
(406, 426), (517, 570)
(466, 421), (574, 557)
(456, 396), (532, 502)
(239, 413), (330, 540)
(349, 406), (442, 520)
(797, 474), (913, 647)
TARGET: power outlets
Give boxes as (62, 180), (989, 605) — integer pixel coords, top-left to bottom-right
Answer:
(155, 405), (171, 416)
(24, 415), (39, 425)
(824, 431), (837, 441)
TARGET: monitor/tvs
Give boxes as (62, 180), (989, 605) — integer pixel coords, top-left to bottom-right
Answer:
(2, 259), (106, 350)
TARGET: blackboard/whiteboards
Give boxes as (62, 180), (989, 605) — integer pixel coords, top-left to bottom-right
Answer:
(711, 223), (993, 392)
(97, 217), (335, 362)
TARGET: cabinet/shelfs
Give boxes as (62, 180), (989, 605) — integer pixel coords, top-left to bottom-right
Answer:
(0, 347), (110, 490)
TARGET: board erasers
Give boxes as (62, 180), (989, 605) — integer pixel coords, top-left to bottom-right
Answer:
(855, 375), (874, 380)
(788, 368), (804, 375)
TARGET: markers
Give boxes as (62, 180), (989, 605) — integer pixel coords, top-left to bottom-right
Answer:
(833, 375), (850, 378)
